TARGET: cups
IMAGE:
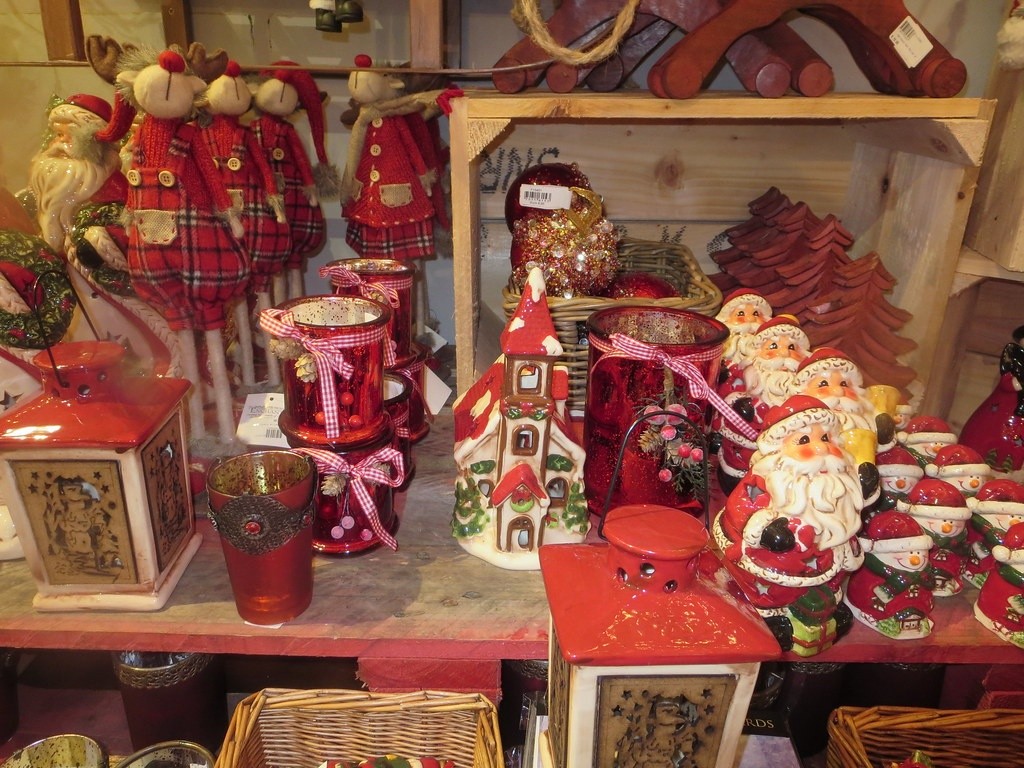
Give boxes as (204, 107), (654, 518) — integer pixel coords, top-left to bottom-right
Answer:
(583, 305), (730, 518)
(204, 257), (430, 626)
(115, 740), (216, 768)
(0, 734), (109, 768)
(112, 652), (228, 756)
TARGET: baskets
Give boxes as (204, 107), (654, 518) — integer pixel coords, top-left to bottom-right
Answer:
(503, 235), (723, 410)
(214, 688), (505, 768)
(826, 706), (1024, 768)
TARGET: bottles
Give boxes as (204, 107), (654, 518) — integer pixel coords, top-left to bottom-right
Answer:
(519, 678), (547, 731)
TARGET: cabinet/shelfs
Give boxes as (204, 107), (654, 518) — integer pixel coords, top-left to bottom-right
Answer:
(0, 0), (1024, 768)
